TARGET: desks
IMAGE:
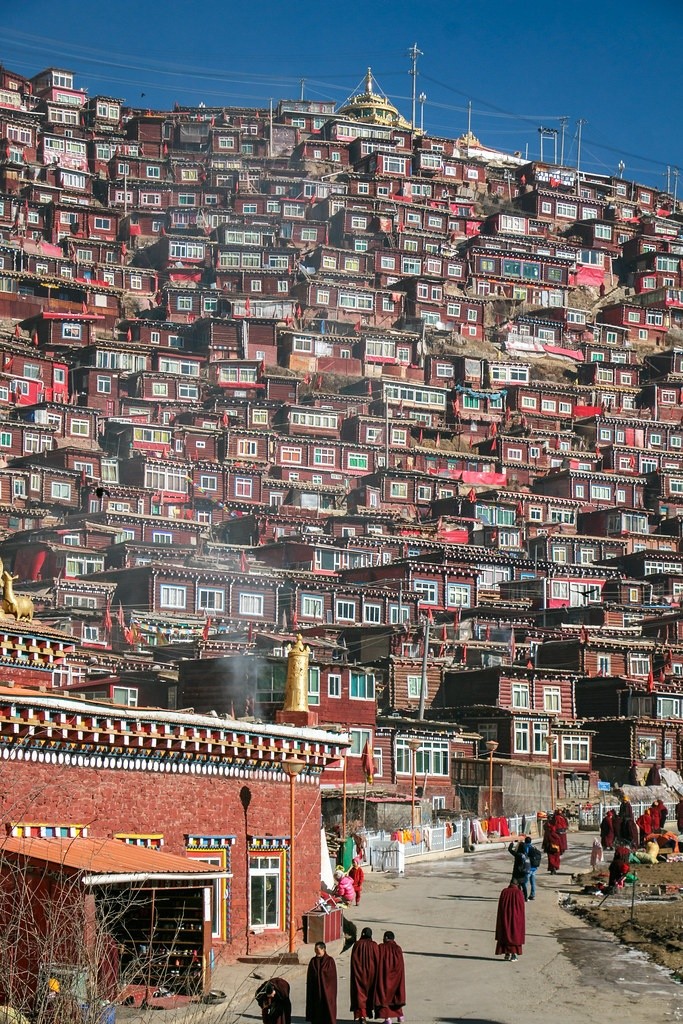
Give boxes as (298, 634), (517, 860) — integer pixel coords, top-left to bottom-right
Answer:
(302, 907), (344, 943)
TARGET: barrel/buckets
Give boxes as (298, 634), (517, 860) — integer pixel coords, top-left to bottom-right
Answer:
(80, 1004), (115, 1024)
(34, 962), (88, 1024)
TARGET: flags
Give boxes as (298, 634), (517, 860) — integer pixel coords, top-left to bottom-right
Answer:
(0, 96), (683, 693)
(362, 739), (374, 786)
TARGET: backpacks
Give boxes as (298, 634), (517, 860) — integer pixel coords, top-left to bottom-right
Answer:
(522, 857), (531, 874)
(527, 846), (541, 867)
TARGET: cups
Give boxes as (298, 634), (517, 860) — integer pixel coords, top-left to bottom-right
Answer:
(139, 923), (202, 978)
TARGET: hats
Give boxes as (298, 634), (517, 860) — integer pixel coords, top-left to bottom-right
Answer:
(50, 978), (60, 993)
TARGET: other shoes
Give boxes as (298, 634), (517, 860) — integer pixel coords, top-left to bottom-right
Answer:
(384, 1019), (392, 1024)
(398, 1016), (404, 1022)
(504, 955), (518, 961)
(163, 922), (204, 931)
(140, 946), (201, 978)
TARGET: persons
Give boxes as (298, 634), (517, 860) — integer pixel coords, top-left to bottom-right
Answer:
(522, 814), (531, 835)
(255, 977), (293, 1024)
(609, 841), (632, 889)
(350, 927), (380, 1024)
(348, 857), (363, 906)
(597, 795), (668, 851)
(600, 283), (606, 296)
(541, 808), (569, 875)
(612, 782), (624, 804)
(375, 931), (406, 1024)
(675, 798), (683, 835)
(645, 761), (661, 786)
(334, 870), (356, 906)
(524, 837), (538, 901)
(306, 941), (337, 1024)
(562, 800), (592, 822)
(494, 878), (526, 962)
(98, 933), (119, 1005)
(509, 841), (528, 902)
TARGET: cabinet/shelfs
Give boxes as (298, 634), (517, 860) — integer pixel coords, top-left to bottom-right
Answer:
(116, 883), (215, 998)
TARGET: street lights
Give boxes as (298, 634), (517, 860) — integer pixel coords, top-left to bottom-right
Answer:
(408, 737), (423, 828)
(485, 739), (499, 816)
(544, 735), (557, 808)
(281, 755), (307, 952)
